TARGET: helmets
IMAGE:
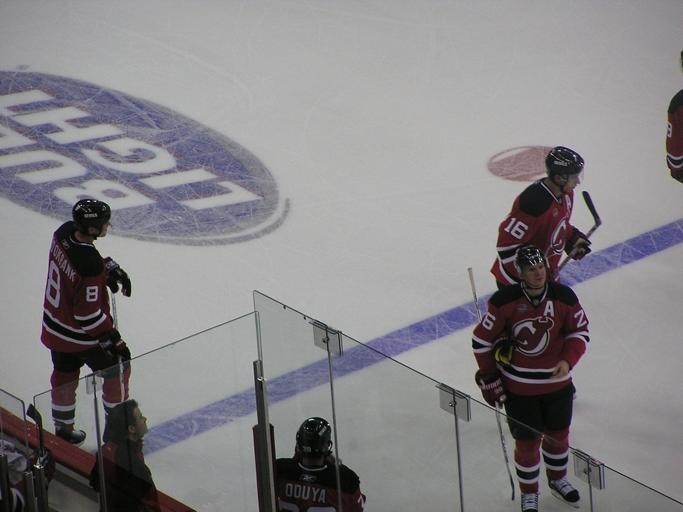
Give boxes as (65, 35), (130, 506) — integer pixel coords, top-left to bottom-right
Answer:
(71, 197), (112, 241)
(513, 244), (550, 290)
(545, 145), (586, 195)
(294, 416), (333, 459)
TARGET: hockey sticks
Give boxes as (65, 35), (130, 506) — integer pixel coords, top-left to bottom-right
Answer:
(468, 267), (515, 500)
(560, 191), (601, 269)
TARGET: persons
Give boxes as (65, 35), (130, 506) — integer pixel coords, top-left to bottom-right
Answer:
(40, 198), (132, 446)
(274, 417), (366, 512)
(0, 435), (56, 512)
(665, 51), (683, 185)
(471, 244), (591, 512)
(89, 399), (162, 512)
(490, 145), (592, 398)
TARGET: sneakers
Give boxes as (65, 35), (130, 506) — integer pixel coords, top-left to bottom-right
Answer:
(520, 493), (539, 512)
(548, 477), (581, 502)
(54, 428), (86, 444)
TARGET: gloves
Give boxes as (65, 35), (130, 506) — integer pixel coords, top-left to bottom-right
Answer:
(104, 256), (132, 297)
(564, 227), (592, 261)
(476, 373), (507, 410)
